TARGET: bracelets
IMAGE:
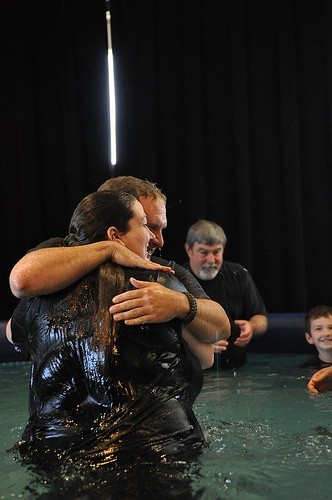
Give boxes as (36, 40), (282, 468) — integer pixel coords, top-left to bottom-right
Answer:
(175, 289), (197, 326)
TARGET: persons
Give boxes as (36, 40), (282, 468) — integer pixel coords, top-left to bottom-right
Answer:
(4, 188), (216, 500)
(181, 219), (269, 372)
(8, 176), (232, 345)
(301, 305), (332, 371)
(306, 365), (332, 397)
